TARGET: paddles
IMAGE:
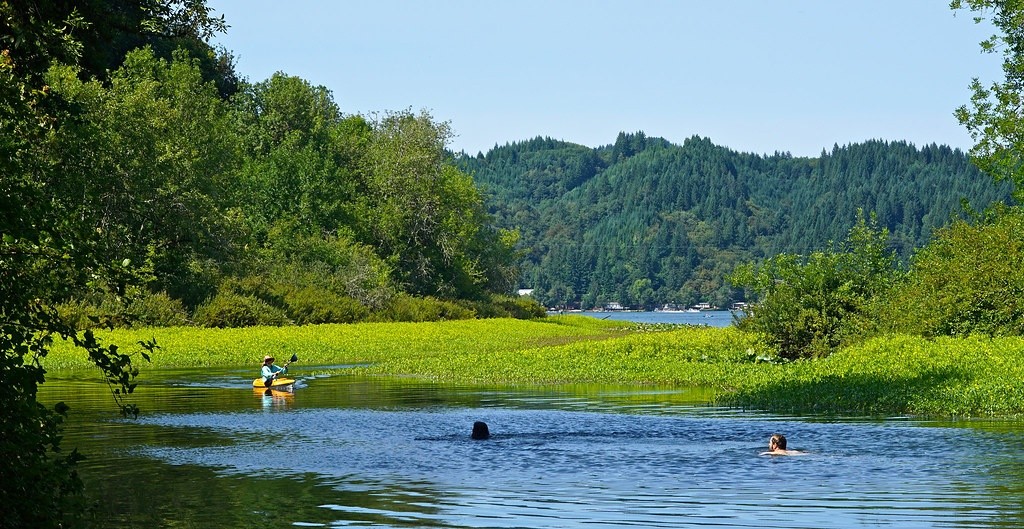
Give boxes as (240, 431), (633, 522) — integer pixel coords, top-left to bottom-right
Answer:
(264, 354), (298, 387)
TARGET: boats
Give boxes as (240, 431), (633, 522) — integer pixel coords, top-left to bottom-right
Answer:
(253, 377), (296, 390)
(656, 303), (701, 314)
(546, 307), (583, 315)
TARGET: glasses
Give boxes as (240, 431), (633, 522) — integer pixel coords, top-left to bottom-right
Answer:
(266, 359), (273, 361)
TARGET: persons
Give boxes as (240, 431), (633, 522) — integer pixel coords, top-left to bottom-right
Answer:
(760, 434), (803, 455)
(261, 355), (288, 384)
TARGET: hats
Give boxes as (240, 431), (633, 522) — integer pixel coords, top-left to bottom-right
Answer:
(261, 355), (275, 366)
